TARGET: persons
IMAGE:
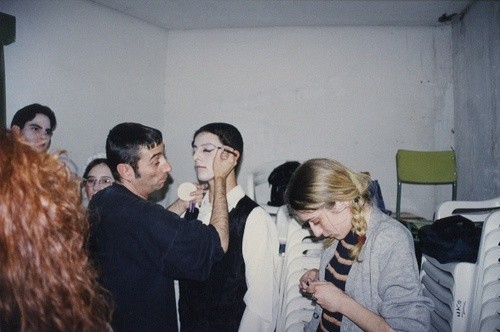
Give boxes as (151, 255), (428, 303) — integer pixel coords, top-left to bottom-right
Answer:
(81, 158), (117, 202)
(284, 157), (436, 332)
(258, 161), (302, 225)
(173, 123), (281, 332)
(11, 103), (57, 153)
(0, 126), (116, 332)
(85, 121), (240, 332)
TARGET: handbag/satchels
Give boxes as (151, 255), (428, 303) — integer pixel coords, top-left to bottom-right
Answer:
(417, 215), (481, 264)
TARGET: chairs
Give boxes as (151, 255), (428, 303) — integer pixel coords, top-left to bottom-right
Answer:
(247, 160), (287, 216)
(417, 196), (500, 332)
(395, 148), (459, 242)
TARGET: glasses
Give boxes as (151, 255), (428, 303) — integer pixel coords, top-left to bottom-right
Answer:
(86, 176), (113, 188)
